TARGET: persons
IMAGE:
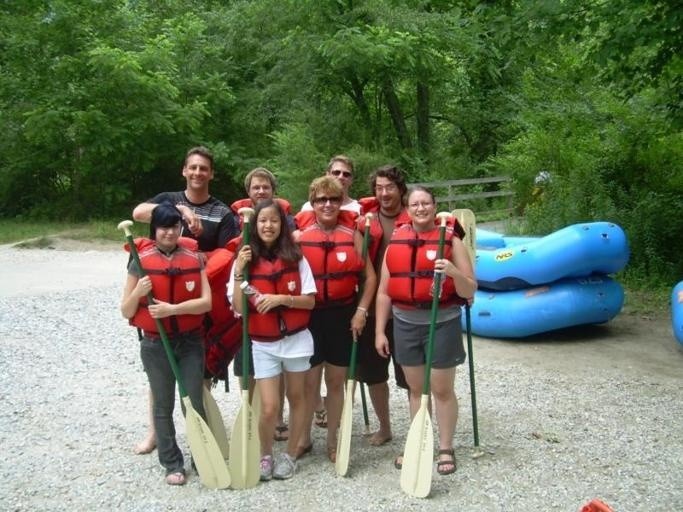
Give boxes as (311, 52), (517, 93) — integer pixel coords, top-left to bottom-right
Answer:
(229, 165), (297, 443)
(120, 202), (213, 484)
(131, 145), (236, 454)
(298, 154), (364, 429)
(226, 197), (319, 482)
(356, 162), (417, 449)
(373, 184), (478, 477)
(289, 174), (379, 463)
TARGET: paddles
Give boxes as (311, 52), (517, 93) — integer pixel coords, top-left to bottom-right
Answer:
(202, 379), (230, 460)
(335, 212), (374, 478)
(452, 209), (484, 460)
(400, 210), (451, 497)
(118, 220), (230, 489)
(230, 206), (261, 489)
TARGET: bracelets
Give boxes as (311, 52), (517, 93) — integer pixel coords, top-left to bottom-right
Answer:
(234, 273), (244, 278)
(356, 306), (369, 318)
(287, 293), (295, 309)
(234, 278), (244, 282)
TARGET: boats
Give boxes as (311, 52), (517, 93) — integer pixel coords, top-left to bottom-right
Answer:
(670, 278), (682, 345)
(460, 276), (624, 338)
(461, 221), (630, 290)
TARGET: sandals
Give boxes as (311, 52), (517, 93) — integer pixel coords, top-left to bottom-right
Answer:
(395, 452), (404, 469)
(437, 449), (456, 475)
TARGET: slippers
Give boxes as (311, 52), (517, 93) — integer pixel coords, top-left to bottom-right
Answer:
(328, 442), (335, 462)
(315, 396), (328, 427)
(273, 424), (290, 440)
(167, 472), (185, 485)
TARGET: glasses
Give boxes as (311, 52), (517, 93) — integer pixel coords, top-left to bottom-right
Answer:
(332, 170), (351, 177)
(376, 184), (395, 190)
(410, 201), (429, 207)
(314, 196), (341, 202)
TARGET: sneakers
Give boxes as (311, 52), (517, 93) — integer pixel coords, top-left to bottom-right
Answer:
(273, 452), (298, 478)
(259, 454), (274, 481)
